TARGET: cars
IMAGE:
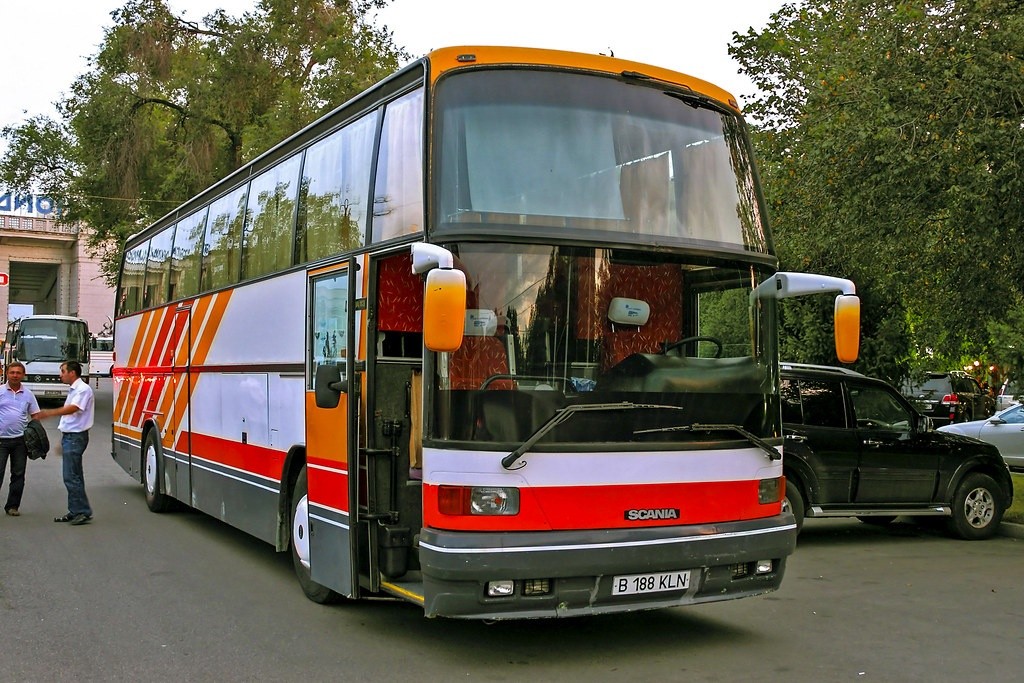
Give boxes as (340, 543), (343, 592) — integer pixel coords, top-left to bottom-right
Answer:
(935, 402), (1024, 467)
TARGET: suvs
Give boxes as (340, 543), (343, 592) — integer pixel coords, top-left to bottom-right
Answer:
(900, 371), (997, 429)
(778, 361), (1013, 541)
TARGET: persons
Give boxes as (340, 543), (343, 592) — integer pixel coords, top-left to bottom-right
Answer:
(0, 362), (41, 516)
(32, 361), (95, 526)
(100, 340), (109, 351)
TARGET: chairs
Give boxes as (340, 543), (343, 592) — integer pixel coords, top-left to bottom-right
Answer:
(448, 308), (517, 390)
(599, 297), (661, 374)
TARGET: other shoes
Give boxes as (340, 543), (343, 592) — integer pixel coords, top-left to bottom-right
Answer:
(7, 509), (19, 516)
(71, 512), (94, 524)
(54, 511), (77, 522)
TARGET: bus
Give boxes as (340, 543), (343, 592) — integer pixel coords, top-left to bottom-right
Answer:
(110, 45), (859, 626)
(87, 334), (114, 377)
(2, 314), (98, 408)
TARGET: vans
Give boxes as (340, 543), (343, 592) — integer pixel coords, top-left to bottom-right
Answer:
(994, 394), (1016, 411)
